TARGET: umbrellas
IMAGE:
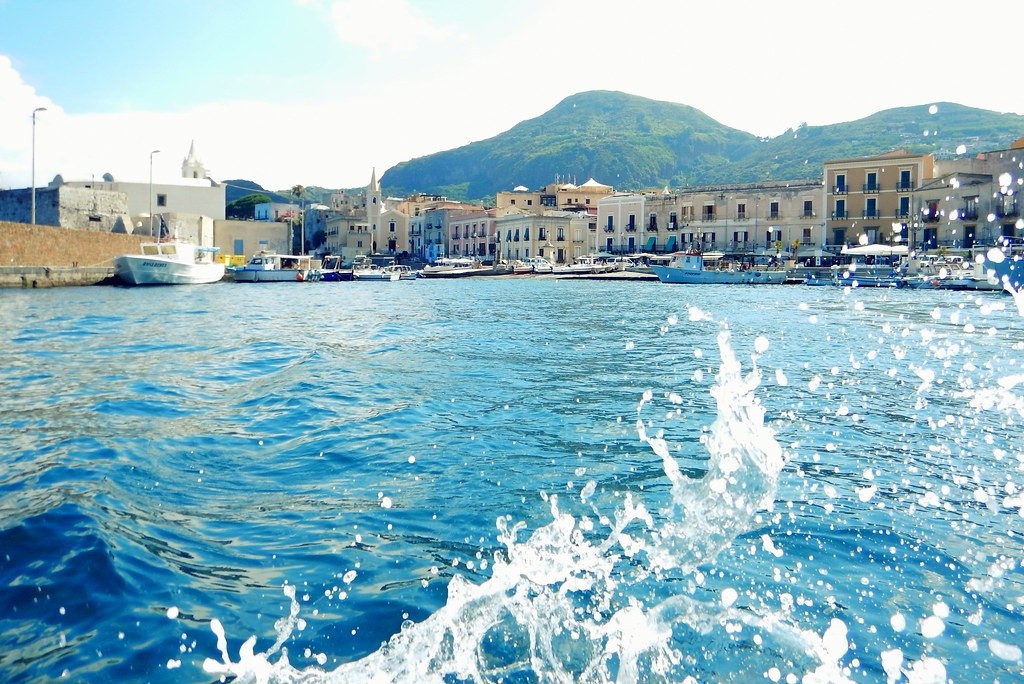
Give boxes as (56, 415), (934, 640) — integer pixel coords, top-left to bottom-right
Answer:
(593, 251), (618, 258)
(796, 247), (836, 257)
(755, 248), (791, 257)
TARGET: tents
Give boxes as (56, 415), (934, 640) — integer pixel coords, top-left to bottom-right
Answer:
(841, 245), (908, 267)
(435, 258), (474, 268)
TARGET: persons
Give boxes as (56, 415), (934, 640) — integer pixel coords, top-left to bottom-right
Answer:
(804, 257), (839, 267)
(734, 256), (776, 272)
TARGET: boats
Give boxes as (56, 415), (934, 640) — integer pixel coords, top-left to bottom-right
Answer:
(422, 252), (655, 280)
(228, 250), (312, 281)
(113, 242), (225, 285)
(789, 241), (1024, 292)
(310, 255), (354, 282)
(649, 252), (787, 285)
(352, 253), (417, 281)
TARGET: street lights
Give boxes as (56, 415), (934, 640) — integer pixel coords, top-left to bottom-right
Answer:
(148, 149), (161, 236)
(30, 106), (47, 226)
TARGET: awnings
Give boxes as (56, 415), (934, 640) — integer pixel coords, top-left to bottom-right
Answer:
(645, 236), (656, 251)
(665, 236), (676, 251)
